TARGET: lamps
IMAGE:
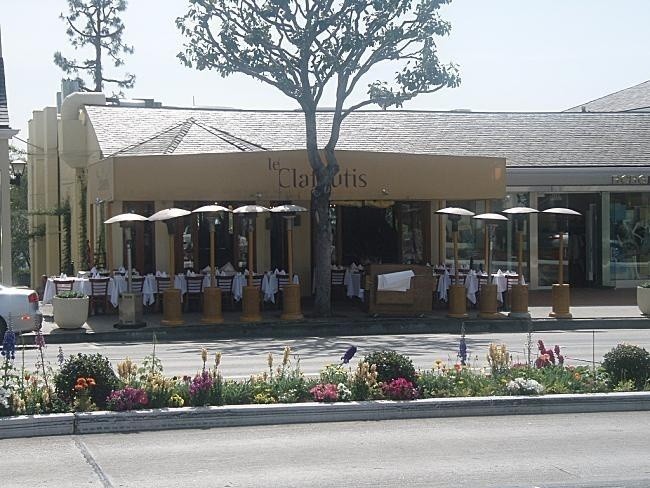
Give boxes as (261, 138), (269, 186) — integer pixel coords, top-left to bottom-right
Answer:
(10, 158), (27, 188)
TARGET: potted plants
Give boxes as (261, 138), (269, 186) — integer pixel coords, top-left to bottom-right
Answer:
(636, 283), (650, 316)
(53, 291), (90, 329)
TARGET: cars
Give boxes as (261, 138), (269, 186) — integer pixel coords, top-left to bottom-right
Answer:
(0, 280), (45, 351)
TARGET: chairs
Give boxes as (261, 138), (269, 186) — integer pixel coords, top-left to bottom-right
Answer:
(327, 267), (520, 310)
(40, 270), (294, 316)
